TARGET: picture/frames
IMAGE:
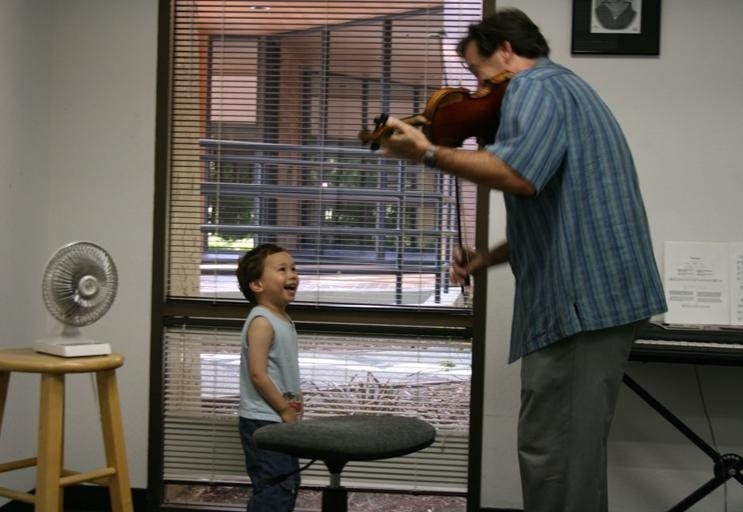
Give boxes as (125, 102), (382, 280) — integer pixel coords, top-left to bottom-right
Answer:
(569, 2), (664, 59)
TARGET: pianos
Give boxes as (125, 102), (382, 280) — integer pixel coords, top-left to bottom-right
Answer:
(628, 320), (743, 367)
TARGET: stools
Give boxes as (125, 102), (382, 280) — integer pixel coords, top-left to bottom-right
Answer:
(250, 415), (437, 510)
(0, 348), (133, 511)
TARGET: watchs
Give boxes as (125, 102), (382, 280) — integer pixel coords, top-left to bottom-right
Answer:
(419, 144), (439, 169)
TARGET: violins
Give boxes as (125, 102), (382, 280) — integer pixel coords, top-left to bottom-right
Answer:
(370, 70), (516, 150)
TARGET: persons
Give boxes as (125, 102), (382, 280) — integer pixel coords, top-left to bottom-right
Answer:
(377, 8), (670, 509)
(233, 242), (305, 512)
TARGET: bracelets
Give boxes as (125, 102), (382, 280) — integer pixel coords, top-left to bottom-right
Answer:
(485, 251), (495, 267)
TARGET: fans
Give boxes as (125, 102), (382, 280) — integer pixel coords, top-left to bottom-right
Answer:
(31, 240), (118, 358)
